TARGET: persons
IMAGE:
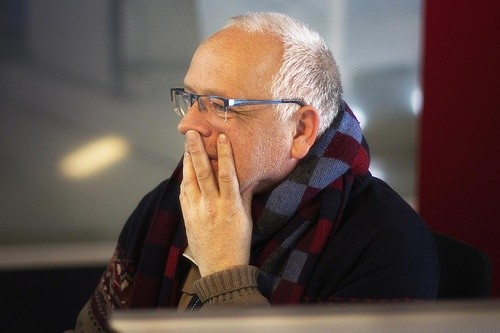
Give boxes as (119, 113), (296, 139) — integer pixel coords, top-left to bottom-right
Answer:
(74, 12), (440, 333)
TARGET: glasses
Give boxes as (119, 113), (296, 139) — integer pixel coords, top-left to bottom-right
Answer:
(170, 88), (305, 127)
